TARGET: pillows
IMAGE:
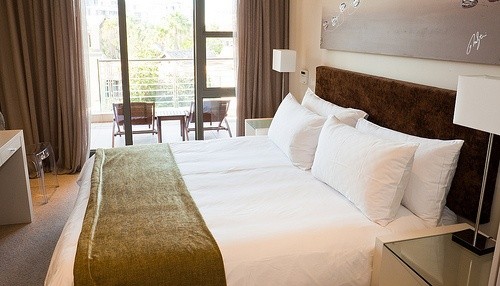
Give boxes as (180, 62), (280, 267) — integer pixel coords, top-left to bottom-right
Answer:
(265, 85), (466, 229)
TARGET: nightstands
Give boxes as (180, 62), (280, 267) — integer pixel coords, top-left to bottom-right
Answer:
(371, 222), (500, 286)
(244, 116), (275, 137)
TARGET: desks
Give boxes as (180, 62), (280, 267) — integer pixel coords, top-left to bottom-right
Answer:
(155, 108), (187, 144)
(0, 127), (33, 226)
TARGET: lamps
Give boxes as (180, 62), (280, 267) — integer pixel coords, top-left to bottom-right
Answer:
(452, 71), (500, 258)
(271, 47), (297, 102)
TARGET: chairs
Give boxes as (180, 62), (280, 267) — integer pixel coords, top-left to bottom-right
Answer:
(186, 99), (233, 141)
(0, 111), (60, 206)
(109, 99), (158, 149)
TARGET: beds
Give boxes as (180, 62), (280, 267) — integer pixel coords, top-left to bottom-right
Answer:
(44, 65), (500, 286)
